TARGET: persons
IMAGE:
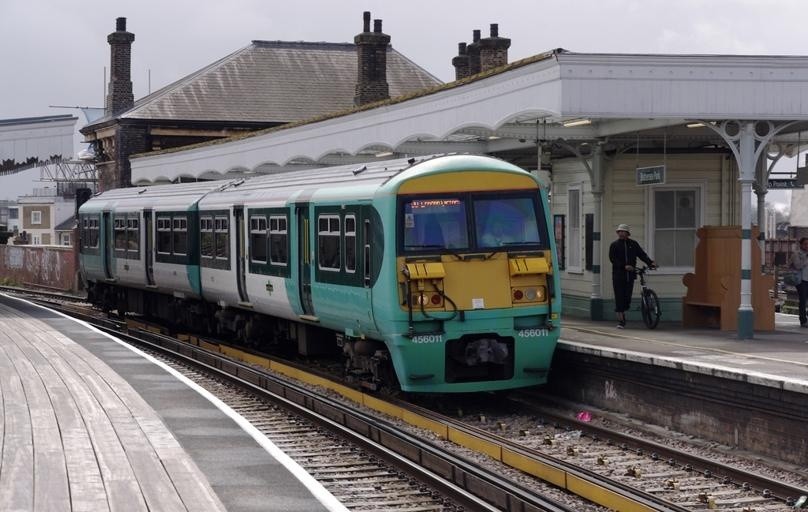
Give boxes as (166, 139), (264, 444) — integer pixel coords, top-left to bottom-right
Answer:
(787, 237), (808, 328)
(609, 224), (657, 329)
(482, 219), (514, 247)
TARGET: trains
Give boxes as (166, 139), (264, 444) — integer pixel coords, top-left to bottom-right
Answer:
(74, 149), (561, 396)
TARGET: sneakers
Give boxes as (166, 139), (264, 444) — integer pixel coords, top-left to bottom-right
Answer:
(616, 320), (626, 329)
(800, 322), (808, 328)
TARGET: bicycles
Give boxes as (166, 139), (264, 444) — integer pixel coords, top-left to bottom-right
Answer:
(625, 261), (662, 329)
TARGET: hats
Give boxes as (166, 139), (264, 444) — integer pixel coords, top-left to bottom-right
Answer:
(616, 224), (631, 236)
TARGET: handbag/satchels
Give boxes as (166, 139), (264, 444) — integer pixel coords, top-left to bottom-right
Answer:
(783, 269), (801, 285)
